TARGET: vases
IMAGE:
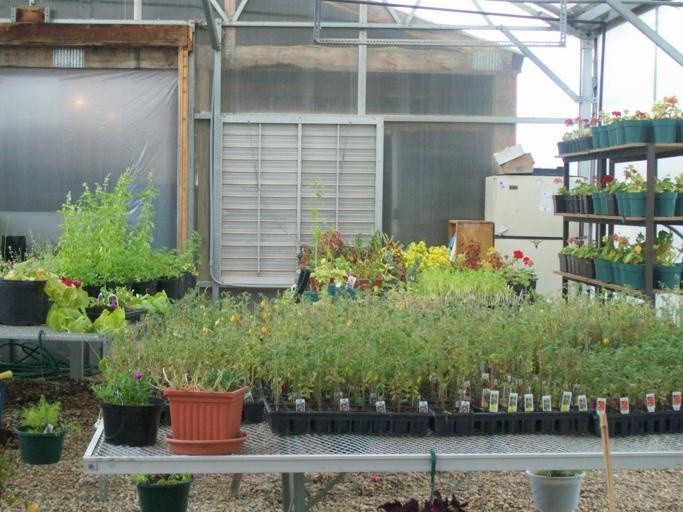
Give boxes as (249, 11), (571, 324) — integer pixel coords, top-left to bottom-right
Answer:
(557, 135), (593, 155)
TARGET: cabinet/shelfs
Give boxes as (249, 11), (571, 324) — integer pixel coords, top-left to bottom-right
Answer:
(553, 142), (683, 316)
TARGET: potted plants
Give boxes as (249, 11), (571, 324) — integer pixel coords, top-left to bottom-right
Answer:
(525, 470), (585, 512)
(131, 473), (194, 512)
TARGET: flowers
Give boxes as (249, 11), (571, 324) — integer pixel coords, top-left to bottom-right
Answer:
(561, 96), (683, 140)
